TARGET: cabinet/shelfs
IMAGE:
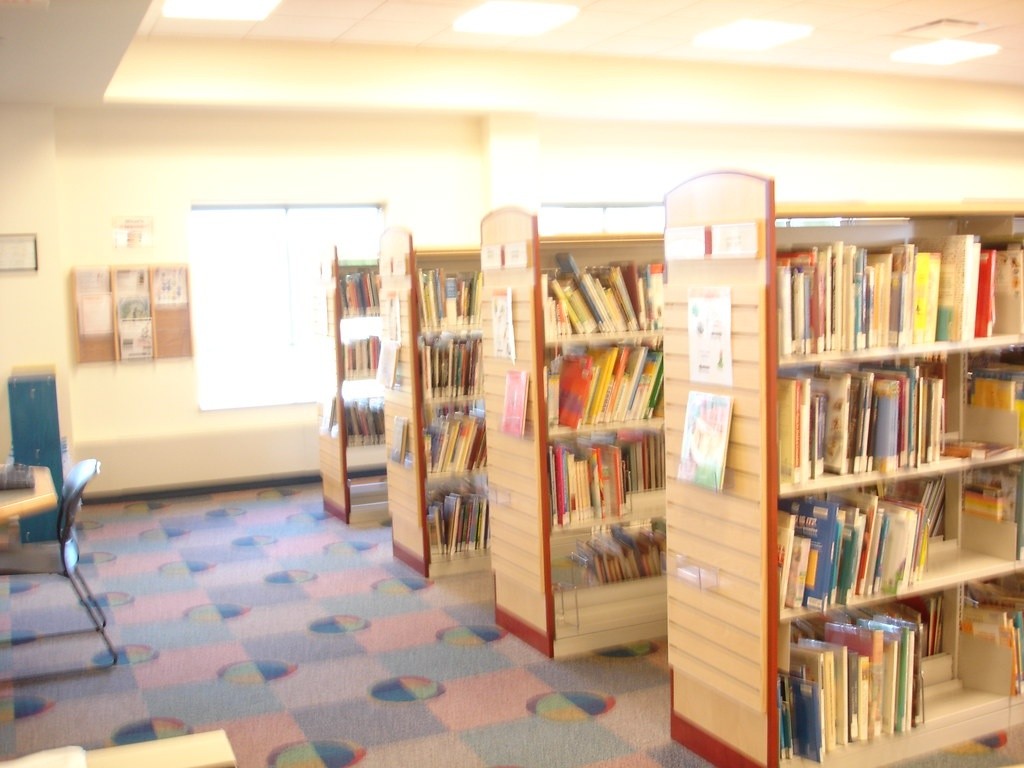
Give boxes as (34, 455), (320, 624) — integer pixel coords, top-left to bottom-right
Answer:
(317, 172), (1024, 768)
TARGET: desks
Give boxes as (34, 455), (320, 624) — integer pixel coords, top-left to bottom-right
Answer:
(0, 464), (59, 528)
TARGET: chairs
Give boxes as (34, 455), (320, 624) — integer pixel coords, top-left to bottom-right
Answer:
(0, 459), (118, 667)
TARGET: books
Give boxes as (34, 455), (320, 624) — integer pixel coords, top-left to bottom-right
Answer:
(340, 273), (384, 446)
(390, 416), (408, 463)
(541, 253), (665, 585)
(677, 391), (733, 491)
(415, 268), (491, 555)
(778, 235), (1024, 764)
(503, 372), (529, 436)
